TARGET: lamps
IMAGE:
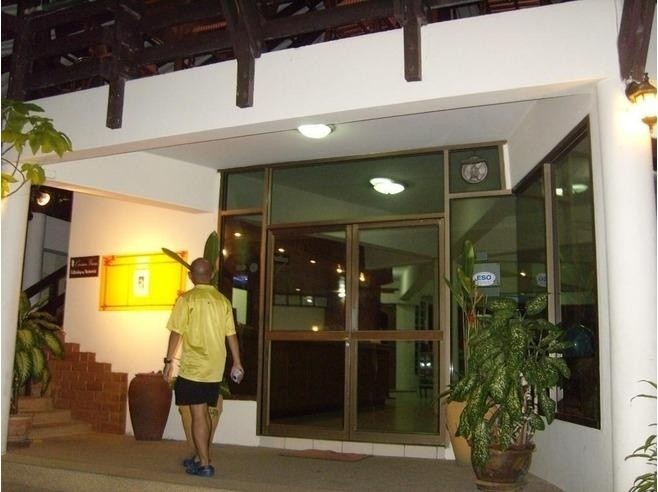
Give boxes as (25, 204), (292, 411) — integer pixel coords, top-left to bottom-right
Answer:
(623, 70), (656, 132)
(296, 124), (335, 138)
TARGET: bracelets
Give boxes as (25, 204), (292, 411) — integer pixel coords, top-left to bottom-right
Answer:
(163, 356), (172, 364)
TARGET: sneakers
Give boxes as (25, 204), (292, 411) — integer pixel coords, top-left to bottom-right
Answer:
(186, 465), (215, 477)
(183, 456), (211, 466)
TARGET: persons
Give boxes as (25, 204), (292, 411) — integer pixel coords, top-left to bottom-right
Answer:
(162, 257), (246, 476)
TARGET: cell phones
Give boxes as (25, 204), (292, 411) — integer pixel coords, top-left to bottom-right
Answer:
(233, 370), (242, 382)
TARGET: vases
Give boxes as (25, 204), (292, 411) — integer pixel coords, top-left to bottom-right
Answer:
(128, 373), (171, 442)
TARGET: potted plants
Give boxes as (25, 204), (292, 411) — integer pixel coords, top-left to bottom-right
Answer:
(7, 291), (66, 449)
(439, 238), (575, 492)
(160, 231), (232, 449)
